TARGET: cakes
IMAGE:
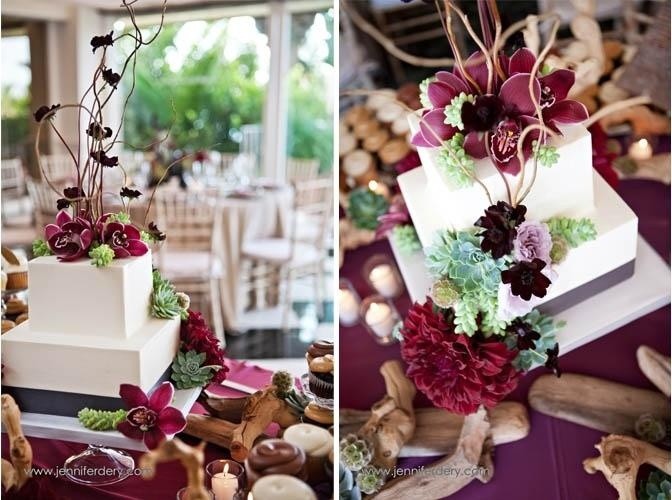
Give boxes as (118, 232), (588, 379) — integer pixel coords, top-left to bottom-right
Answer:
(1, 0), (230, 451)
(373, 0), (640, 416)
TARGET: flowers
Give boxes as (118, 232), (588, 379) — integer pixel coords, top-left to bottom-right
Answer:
(411, 48), (588, 175)
(118, 381), (188, 452)
(181, 309), (231, 385)
(400, 201), (560, 416)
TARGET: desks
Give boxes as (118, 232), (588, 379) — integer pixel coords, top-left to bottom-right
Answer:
(339, 76), (671, 500)
(2, 358), (300, 500)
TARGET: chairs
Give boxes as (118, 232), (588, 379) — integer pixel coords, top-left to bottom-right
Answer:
(1, 126), (331, 351)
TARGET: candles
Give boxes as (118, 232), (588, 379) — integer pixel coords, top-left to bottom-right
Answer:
(370, 265), (398, 297)
(339, 289), (360, 324)
(211, 463), (238, 500)
(365, 303), (394, 337)
